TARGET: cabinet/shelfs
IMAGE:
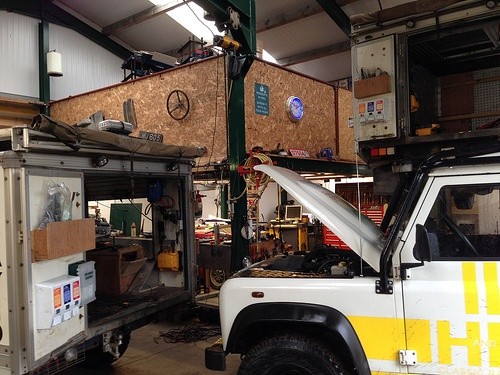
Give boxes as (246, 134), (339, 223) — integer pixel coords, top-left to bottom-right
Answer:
(87, 247), (148, 297)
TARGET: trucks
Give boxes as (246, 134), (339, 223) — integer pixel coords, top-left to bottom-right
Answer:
(0, 118), (200, 375)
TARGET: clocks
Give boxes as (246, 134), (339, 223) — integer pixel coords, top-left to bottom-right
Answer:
(286, 96), (303, 122)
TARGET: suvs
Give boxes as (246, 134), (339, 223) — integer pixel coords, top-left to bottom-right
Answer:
(201, 133), (499, 375)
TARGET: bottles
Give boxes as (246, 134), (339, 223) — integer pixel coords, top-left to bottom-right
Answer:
(131, 223), (136, 236)
